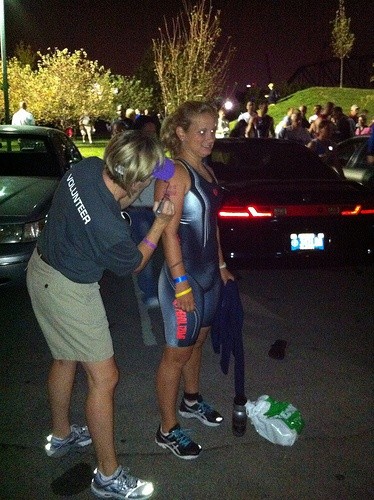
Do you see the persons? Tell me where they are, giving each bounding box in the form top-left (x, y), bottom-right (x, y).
top-left (215, 82), bottom-right (373, 155)
top-left (26, 130), bottom-right (177, 499)
top-left (80, 110), bottom-right (96, 144)
top-left (110, 104), bottom-right (162, 133)
top-left (12, 101), bottom-right (35, 126)
top-left (129, 116), bottom-right (161, 306)
top-left (156, 101), bottom-right (237, 460)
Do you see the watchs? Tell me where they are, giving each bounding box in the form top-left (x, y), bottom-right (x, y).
top-left (219, 262), bottom-right (227, 270)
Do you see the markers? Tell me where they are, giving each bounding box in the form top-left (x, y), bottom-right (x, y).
top-left (156, 194), bottom-right (169, 216)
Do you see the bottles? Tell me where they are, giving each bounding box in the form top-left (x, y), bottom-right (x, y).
top-left (232, 393), bottom-right (247, 437)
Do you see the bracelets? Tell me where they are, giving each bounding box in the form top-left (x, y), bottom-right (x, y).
top-left (142, 237), bottom-right (158, 249)
top-left (169, 259), bottom-right (183, 270)
top-left (171, 275), bottom-right (188, 284)
top-left (174, 287), bottom-right (192, 298)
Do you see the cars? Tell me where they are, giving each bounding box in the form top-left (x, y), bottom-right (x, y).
top-left (333, 134), bottom-right (374, 184)
top-left (202, 136), bottom-right (374, 270)
top-left (0, 124), bottom-right (82, 287)
